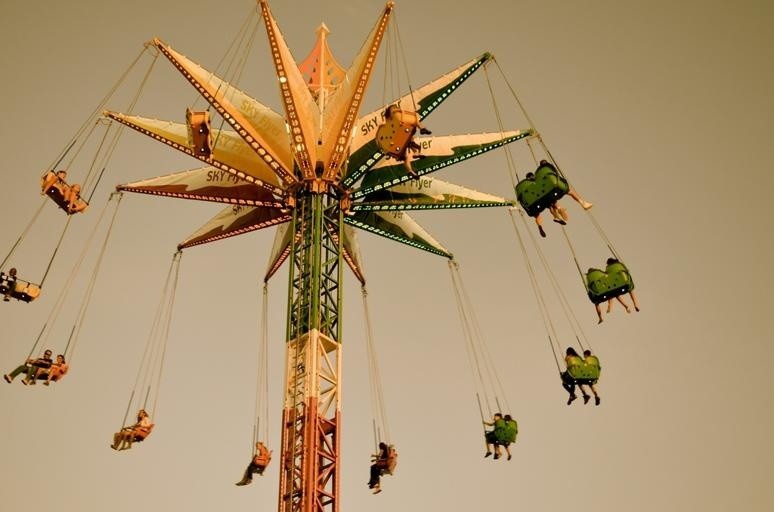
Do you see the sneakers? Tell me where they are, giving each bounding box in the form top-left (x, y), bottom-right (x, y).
top-left (583, 203), bottom-right (592, 210)
top-left (554, 207), bottom-right (568, 224)
top-left (408, 172), bottom-right (419, 179)
top-left (411, 142), bottom-right (421, 148)
top-left (568, 396), bottom-right (600, 405)
top-left (22, 380), bottom-right (28, 385)
top-left (485, 452), bottom-right (498, 459)
top-left (421, 129), bottom-right (432, 134)
top-left (538, 225), bottom-right (546, 237)
top-left (4, 374), bottom-right (12, 383)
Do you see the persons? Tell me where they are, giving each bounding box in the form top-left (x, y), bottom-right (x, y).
top-left (399, 145), bottom-right (426, 181)
top-left (577, 349), bottom-right (601, 406)
top-left (523, 172), bottom-right (567, 238)
top-left (539, 159), bottom-right (592, 221)
top-left (371, 445), bottom-right (396, 494)
top-left (560, 346), bottom-right (582, 406)
top-left (234, 441), bottom-right (268, 486)
top-left (28, 354), bottom-right (68, 386)
top-left (66, 184), bottom-right (88, 215)
top-left (39, 170), bottom-right (71, 208)
top-left (383, 104), bottom-right (432, 150)
top-left (0, 267), bottom-right (16, 302)
top-left (482, 412), bottom-right (505, 459)
top-left (584, 267), bottom-right (612, 325)
top-left (495, 414), bottom-right (517, 460)
top-left (3, 349), bottom-right (52, 385)
top-left (109, 409), bottom-right (149, 451)
top-left (605, 257), bottom-right (639, 313)
top-left (366, 442), bottom-right (391, 489)
